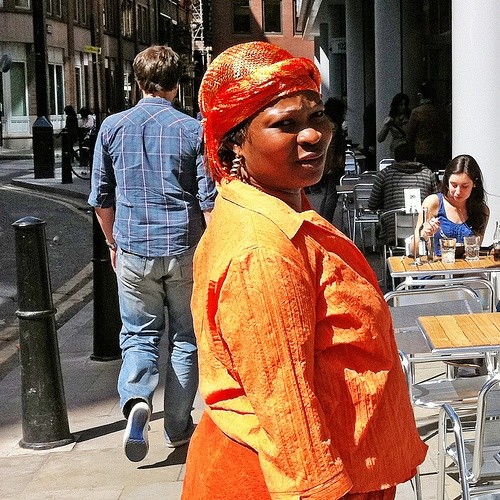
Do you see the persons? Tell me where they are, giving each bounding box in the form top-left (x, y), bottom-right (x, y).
top-left (368, 143), bottom-right (439, 291)
top-left (319, 97), bottom-right (348, 225)
top-left (62, 106), bottom-right (96, 167)
top-left (410, 155), bottom-right (490, 289)
top-left (180, 42), bottom-right (428, 500)
top-left (378, 81), bottom-right (440, 172)
top-left (87, 45), bottom-right (218, 462)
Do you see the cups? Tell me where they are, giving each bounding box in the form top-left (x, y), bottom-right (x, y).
top-left (439, 238), bottom-right (456, 264)
top-left (464, 237), bottom-right (480, 261)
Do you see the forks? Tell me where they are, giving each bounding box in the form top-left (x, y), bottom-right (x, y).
top-left (430, 212), bottom-right (447, 239)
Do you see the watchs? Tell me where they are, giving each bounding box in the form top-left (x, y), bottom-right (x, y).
top-left (419, 225), bottom-right (429, 242)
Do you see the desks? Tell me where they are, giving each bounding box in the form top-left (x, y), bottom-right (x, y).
top-left (417, 312), bottom-right (500, 380)
top-left (388, 254), bottom-right (500, 287)
top-left (347, 155), bottom-right (366, 159)
top-left (336, 185), bottom-right (376, 252)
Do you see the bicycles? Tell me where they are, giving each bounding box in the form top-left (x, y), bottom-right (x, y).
top-left (71, 126), bottom-right (95, 180)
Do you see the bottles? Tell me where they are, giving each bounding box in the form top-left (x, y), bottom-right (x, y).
top-left (493, 221), bottom-right (500, 262)
top-left (419, 207), bottom-right (435, 264)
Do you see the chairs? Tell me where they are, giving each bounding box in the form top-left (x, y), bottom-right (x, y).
top-left (340, 149), bottom-right (500, 500)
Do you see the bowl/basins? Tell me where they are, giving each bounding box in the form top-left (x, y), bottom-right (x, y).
top-left (456, 243), bottom-right (465, 258)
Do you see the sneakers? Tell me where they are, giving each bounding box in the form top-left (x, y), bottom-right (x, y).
top-left (124, 401), bottom-right (150, 462)
top-left (166, 423), bottom-right (198, 447)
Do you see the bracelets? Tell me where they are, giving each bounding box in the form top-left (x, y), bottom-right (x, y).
top-left (106, 241), bottom-right (116, 249)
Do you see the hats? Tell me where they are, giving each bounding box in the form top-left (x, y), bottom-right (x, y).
top-left (198, 42), bottom-right (323, 185)
